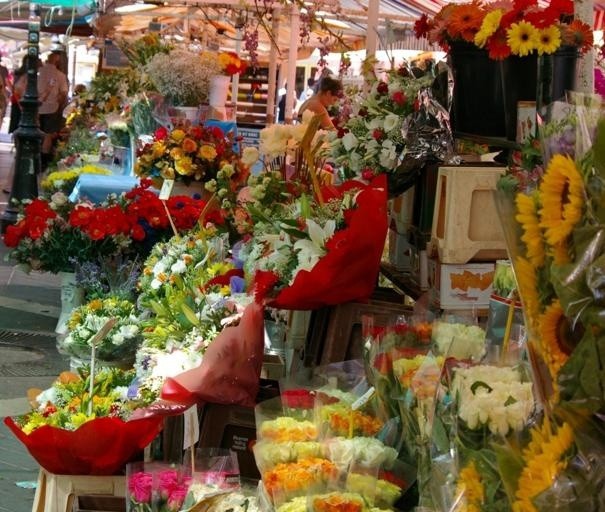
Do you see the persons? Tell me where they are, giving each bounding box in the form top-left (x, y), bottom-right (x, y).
top-left (314, 69), bottom-right (333, 94)
top-left (299, 79), bottom-right (315, 104)
top-left (13, 53), bottom-right (69, 165)
top-left (278, 84), bottom-right (297, 124)
top-left (72, 84), bottom-right (86, 97)
top-left (297, 76), bottom-right (344, 131)
top-left (8, 57), bottom-right (42, 147)
top-left (0, 54), bottom-right (13, 129)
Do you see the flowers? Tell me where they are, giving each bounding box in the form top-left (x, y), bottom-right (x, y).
top-left (5, 1), bottom-right (605, 508)
